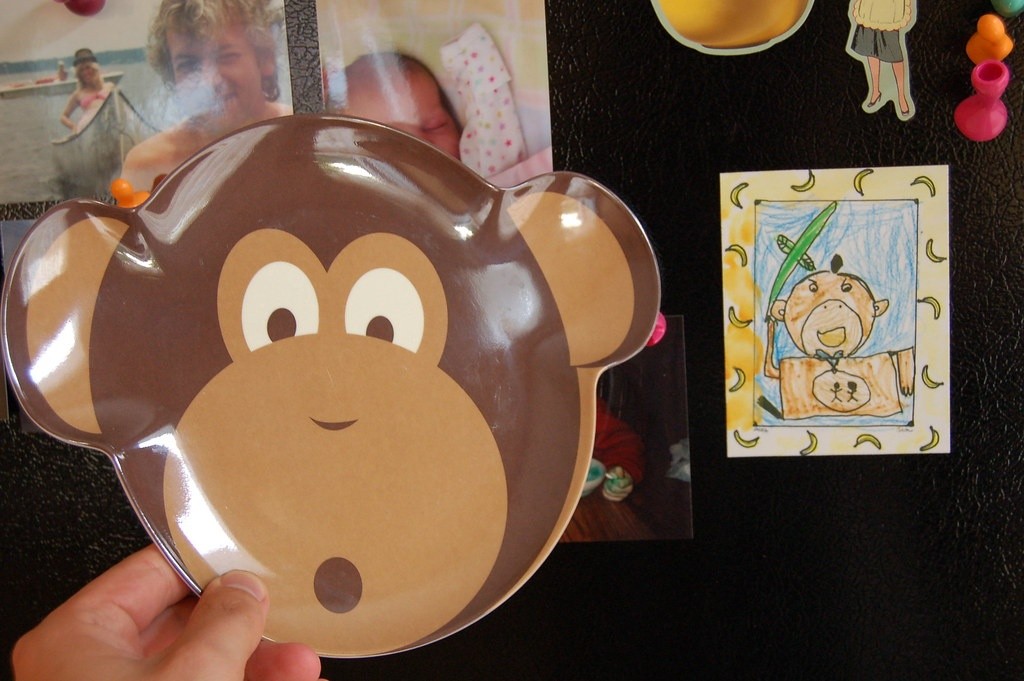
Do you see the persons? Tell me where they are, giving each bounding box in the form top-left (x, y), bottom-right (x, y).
top-left (330, 51), bottom-right (462, 165)
top-left (119, 1), bottom-right (294, 213)
top-left (59, 50), bottom-right (113, 132)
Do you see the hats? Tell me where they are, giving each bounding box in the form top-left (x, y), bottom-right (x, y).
top-left (73, 48), bottom-right (96, 66)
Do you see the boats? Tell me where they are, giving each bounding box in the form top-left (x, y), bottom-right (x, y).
top-left (1, 69), bottom-right (125, 99)
